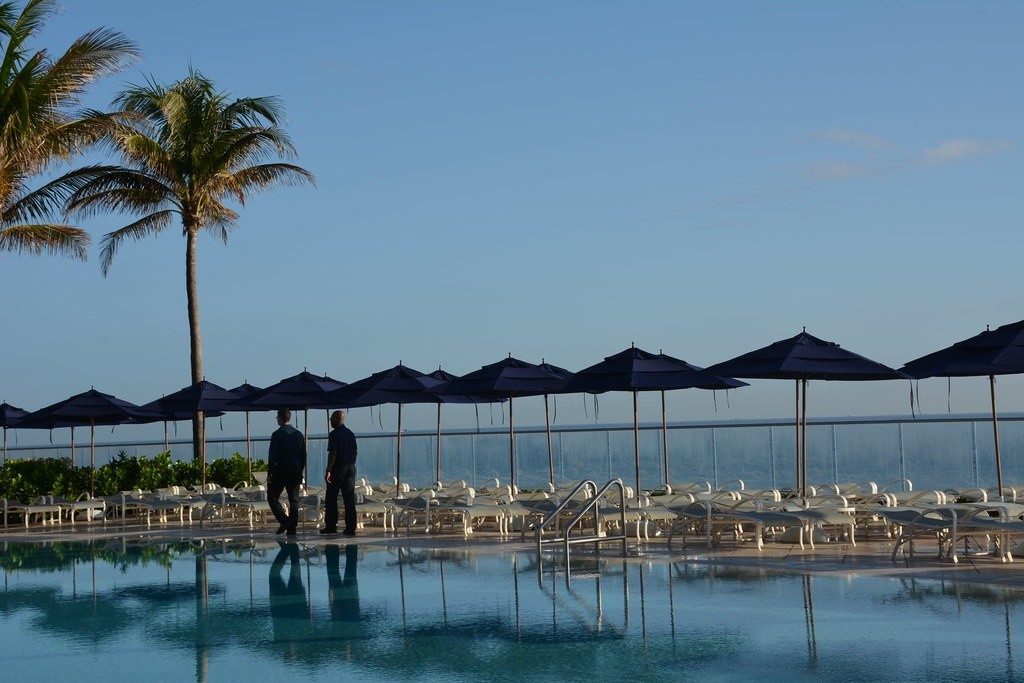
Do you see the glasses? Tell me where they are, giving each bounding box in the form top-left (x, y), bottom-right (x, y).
top-left (275, 417), bottom-right (279, 419)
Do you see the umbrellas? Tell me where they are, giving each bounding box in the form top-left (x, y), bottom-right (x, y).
top-left (0, 321), bottom-right (1024, 506)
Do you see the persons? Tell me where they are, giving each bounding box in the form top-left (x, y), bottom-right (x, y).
top-left (266, 411), bottom-right (307, 537)
top-left (265, 541), bottom-right (361, 646)
top-left (318, 411), bottom-right (358, 536)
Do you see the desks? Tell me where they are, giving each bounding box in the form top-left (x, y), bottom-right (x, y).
top-left (808, 505), bottom-right (856, 544)
top-left (965, 502), bottom-right (1024, 563)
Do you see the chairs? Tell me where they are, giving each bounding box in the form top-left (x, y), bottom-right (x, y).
top-left (0, 477), bottom-right (1024, 567)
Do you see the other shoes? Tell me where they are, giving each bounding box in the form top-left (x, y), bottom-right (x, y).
top-left (343, 529), bottom-right (355, 535)
top-left (277, 541), bottom-right (288, 547)
top-left (320, 527), bottom-right (336, 533)
top-left (276, 517), bottom-right (293, 534)
top-left (286, 528), bottom-right (296, 535)
